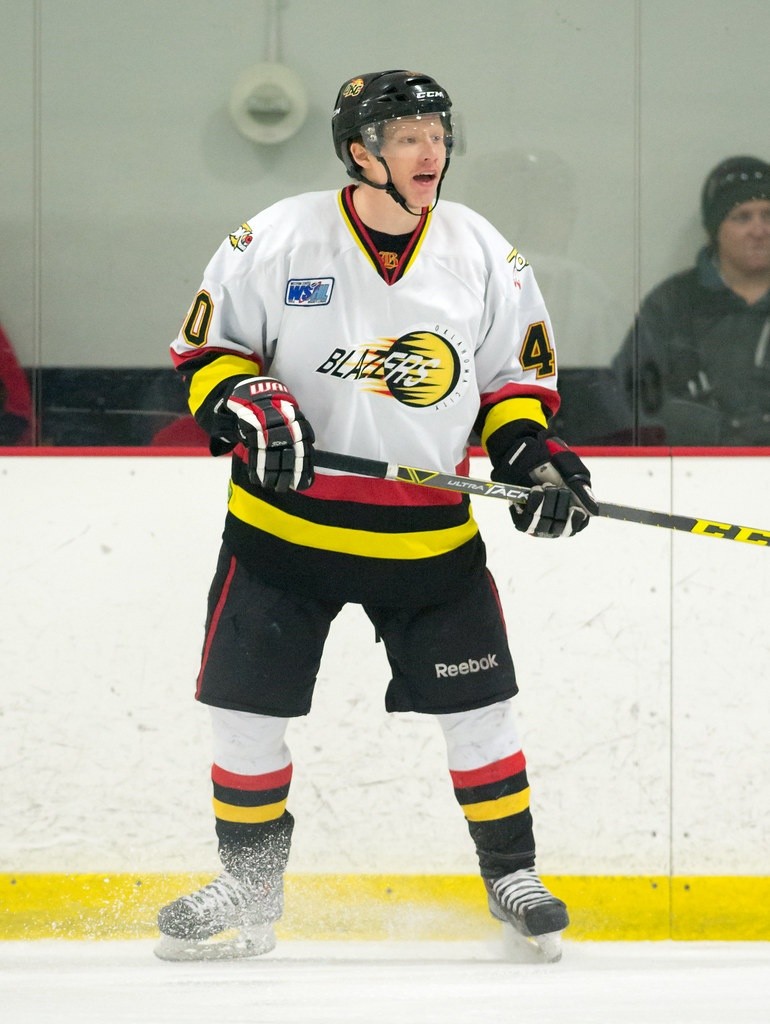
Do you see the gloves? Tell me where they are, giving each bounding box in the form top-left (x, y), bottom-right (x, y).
top-left (491, 428), bottom-right (600, 539)
top-left (224, 376), bottom-right (316, 495)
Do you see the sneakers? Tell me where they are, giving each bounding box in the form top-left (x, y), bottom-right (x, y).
top-left (470, 849), bottom-right (570, 963)
top-left (153, 810), bottom-right (295, 961)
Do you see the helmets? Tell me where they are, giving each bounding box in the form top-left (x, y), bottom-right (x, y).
top-left (331, 67), bottom-right (453, 176)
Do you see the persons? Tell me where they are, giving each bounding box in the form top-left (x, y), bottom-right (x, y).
top-left (152, 69), bottom-right (600, 968)
top-left (593, 149), bottom-right (770, 445)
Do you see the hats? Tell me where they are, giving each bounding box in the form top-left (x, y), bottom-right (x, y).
top-left (701, 155), bottom-right (770, 242)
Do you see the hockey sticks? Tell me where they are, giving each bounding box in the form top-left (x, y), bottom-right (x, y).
top-left (314, 449), bottom-right (770, 548)
